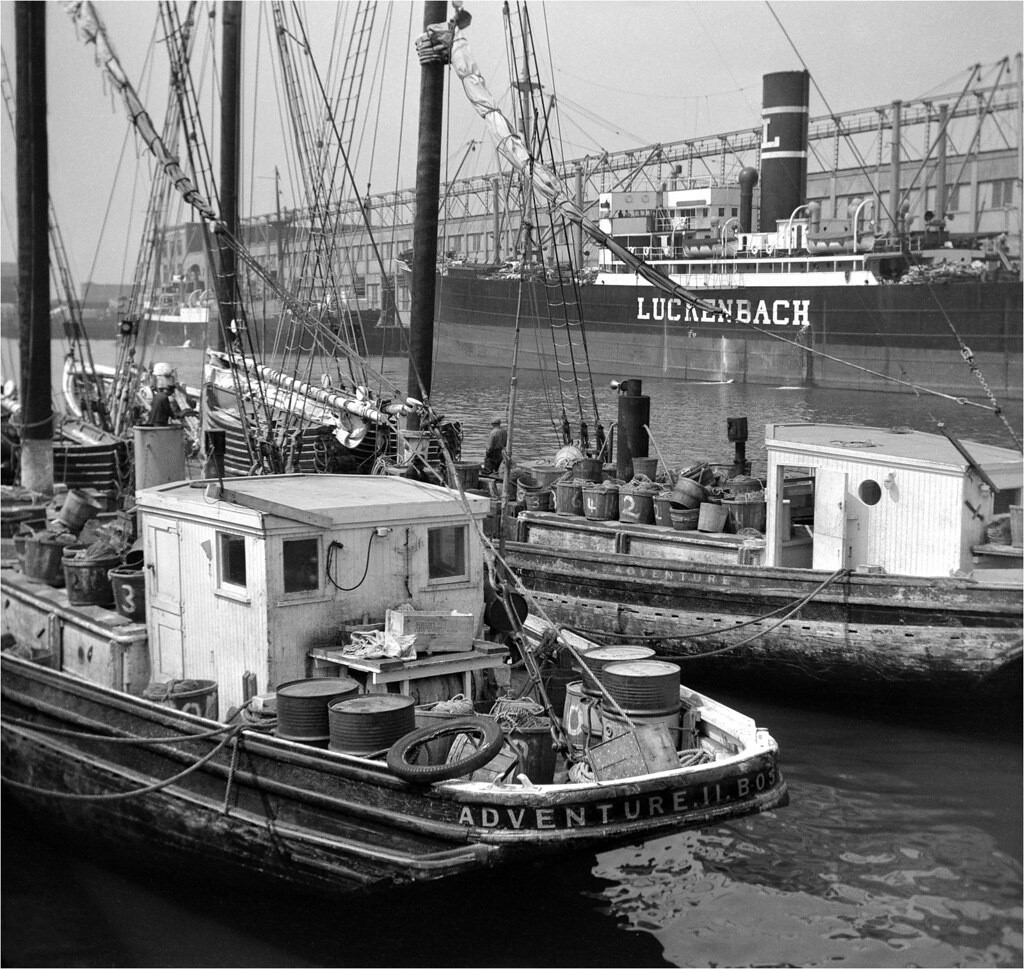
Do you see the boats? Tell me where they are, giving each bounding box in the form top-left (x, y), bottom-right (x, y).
top-left (395, 193), bottom-right (1023, 401)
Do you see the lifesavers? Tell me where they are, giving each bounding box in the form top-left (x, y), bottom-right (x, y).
top-left (384, 711), bottom-right (504, 786)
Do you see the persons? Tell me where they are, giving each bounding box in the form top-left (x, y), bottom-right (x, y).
top-left (147, 386), bottom-right (177, 427)
top-left (484, 419), bottom-right (507, 472)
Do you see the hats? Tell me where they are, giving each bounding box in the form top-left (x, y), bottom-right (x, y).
top-left (167, 385), bottom-right (176, 392)
top-left (491, 419), bottom-right (500, 424)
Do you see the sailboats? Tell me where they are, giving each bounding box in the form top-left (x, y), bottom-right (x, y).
top-left (61, 0), bottom-right (1023, 688)
top-left (1, 0), bottom-right (792, 909)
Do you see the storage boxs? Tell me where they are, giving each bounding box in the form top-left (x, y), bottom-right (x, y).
top-left (585, 721), bottom-right (678, 783)
top-left (386, 608), bottom-right (475, 654)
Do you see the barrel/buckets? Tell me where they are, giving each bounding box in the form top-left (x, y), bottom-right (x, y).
top-left (669, 476), bottom-right (709, 509)
top-left (110, 569), bottom-right (148, 621)
top-left (58, 489), bottom-right (102, 530)
top-left (449, 460), bottom-right (481, 490)
top-left (25, 537), bottom-right (64, 586)
top-left (572, 458), bottom-right (603, 484)
top-left (63, 542), bottom-right (96, 558)
top-left (124, 549), bottom-right (144, 567)
top-left (517, 476), bottom-right (543, 506)
top-left (557, 481), bottom-right (583, 516)
top-left (721, 496), bottom-right (767, 534)
top-left (618, 489), bottom-right (659, 524)
top-left (13, 531), bottom-right (33, 575)
top-left (530, 466), bottom-right (568, 489)
top-left (632, 457), bottom-right (658, 483)
top-left (62, 554), bottom-right (122, 606)
top-left (726, 476), bottom-right (758, 495)
top-left (582, 486), bottom-right (619, 521)
top-left (525, 490), bottom-right (551, 511)
top-left (146, 679), bottom-right (219, 722)
top-left (652, 494), bottom-right (673, 527)
top-left (697, 502), bottom-right (729, 533)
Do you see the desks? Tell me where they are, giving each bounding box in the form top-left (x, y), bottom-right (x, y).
top-left (313, 646), bottom-right (506, 703)
